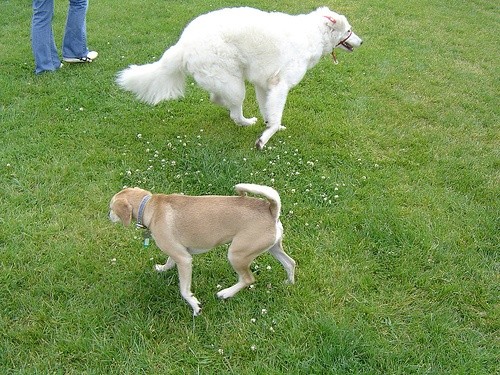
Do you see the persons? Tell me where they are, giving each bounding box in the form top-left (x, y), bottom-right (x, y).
top-left (31, 0), bottom-right (99, 75)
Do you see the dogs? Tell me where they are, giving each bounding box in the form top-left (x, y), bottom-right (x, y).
top-left (112, 6), bottom-right (363, 151)
top-left (108, 183), bottom-right (296, 317)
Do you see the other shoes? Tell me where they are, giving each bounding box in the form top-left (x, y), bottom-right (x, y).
top-left (59, 62), bottom-right (64, 68)
top-left (64, 50), bottom-right (98, 63)
